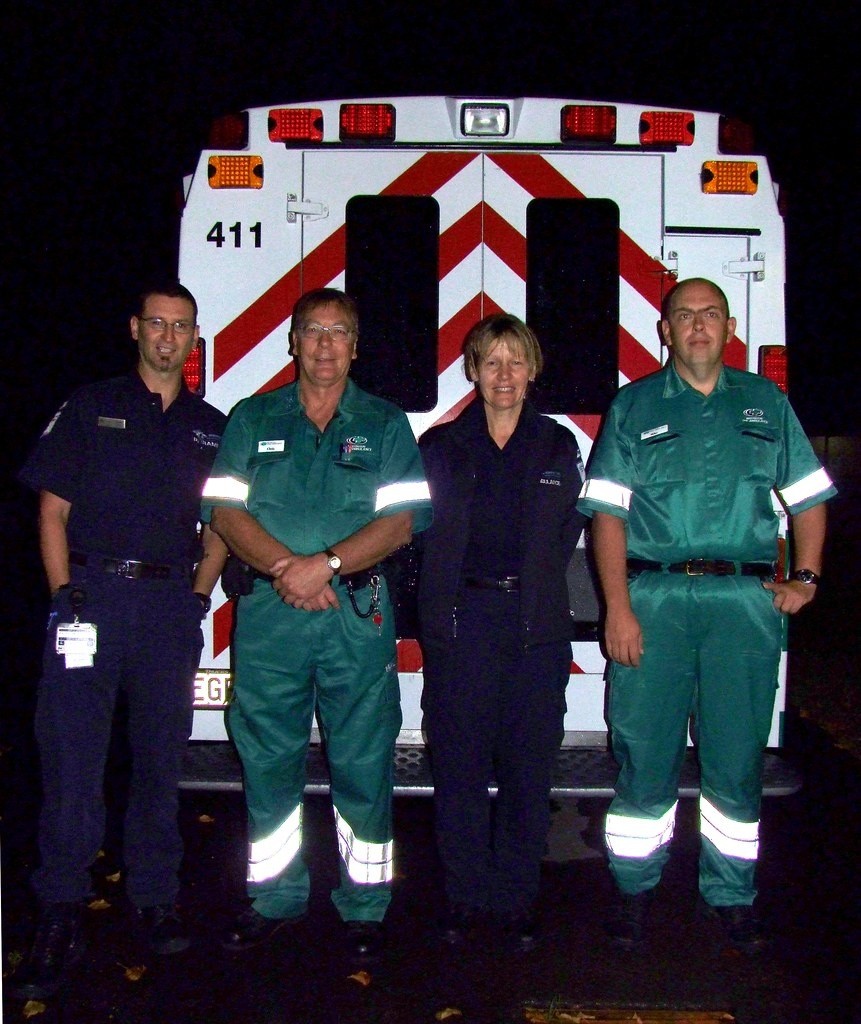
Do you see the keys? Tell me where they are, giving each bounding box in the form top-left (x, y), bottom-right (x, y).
top-left (371, 598), bottom-right (382, 636)
top-left (451, 619), bottom-right (457, 637)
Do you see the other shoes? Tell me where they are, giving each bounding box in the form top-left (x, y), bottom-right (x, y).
top-left (435, 904), bottom-right (485, 948)
top-left (612, 889), bottom-right (654, 944)
top-left (502, 919), bottom-right (549, 965)
top-left (40, 903), bottom-right (85, 953)
top-left (714, 905), bottom-right (763, 942)
top-left (131, 907), bottom-right (195, 957)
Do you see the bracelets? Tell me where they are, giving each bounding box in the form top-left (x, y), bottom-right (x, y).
top-left (195, 593), bottom-right (211, 613)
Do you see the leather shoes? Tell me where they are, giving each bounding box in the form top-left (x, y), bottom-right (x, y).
top-left (218, 909), bottom-right (302, 949)
top-left (346, 920), bottom-right (388, 965)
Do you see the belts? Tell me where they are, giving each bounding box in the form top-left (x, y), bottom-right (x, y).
top-left (252, 565), bottom-right (386, 586)
top-left (625, 557), bottom-right (776, 580)
top-left (67, 549), bottom-right (191, 581)
top-left (459, 575), bottom-right (522, 590)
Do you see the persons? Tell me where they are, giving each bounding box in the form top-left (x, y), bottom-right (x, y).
top-left (15, 283), bottom-right (231, 974)
top-left (576, 277), bottom-right (840, 939)
top-left (401, 313), bottom-right (585, 947)
top-left (200, 287), bottom-right (435, 953)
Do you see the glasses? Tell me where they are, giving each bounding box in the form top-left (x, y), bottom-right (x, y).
top-left (295, 323), bottom-right (358, 340)
top-left (138, 316), bottom-right (198, 334)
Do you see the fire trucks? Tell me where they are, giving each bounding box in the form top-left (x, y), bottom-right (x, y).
top-left (180, 96), bottom-right (803, 799)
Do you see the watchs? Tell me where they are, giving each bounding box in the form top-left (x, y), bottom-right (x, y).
top-left (324, 550), bottom-right (342, 575)
top-left (790, 569), bottom-right (819, 586)
